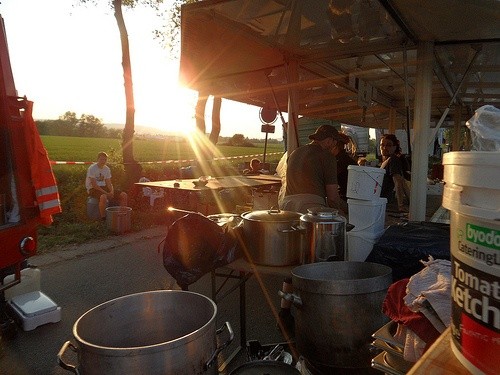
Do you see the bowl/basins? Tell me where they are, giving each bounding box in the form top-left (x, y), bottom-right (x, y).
top-left (192, 181), bottom-right (208, 186)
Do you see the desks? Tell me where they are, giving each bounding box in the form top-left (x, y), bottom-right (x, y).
top-left (211, 246), bottom-right (298, 373)
top-left (135, 174), bottom-right (281, 252)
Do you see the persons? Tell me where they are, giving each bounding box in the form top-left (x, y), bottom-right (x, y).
top-left (379, 134), bottom-right (410, 213)
top-left (333, 133), bottom-right (358, 207)
top-left (246, 158), bottom-right (274, 191)
top-left (357, 157), bottom-right (367, 166)
top-left (85, 152), bottom-right (128, 222)
top-left (278, 124), bottom-right (347, 214)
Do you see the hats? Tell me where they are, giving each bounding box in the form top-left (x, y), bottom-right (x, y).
top-left (308, 123), bottom-right (340, 140)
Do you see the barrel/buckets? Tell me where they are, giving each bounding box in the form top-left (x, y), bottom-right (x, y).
top-left (240, 205), bottom-right (304, 267)
top-left (229, 361), bottom-right (303, 375)
top-left (291, 206), bottom-right (356, 264)
top-left (346, 165), bottom-right (386, 201)
top-left (105, 206), bottom-right (133, 233)
top-left (440, 151), bottom-right (500, 375)
top-left (347, 197), bottom-right (388, 233)
top-left (56, 289), bottom-right (235, 375)
top-left (278, 261), bottom-right (393, 369)
top-left (346, 232), bottom-right (381, 262)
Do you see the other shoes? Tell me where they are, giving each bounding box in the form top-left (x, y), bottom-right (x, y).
top-left (99, 216), bottom-right (106, 224)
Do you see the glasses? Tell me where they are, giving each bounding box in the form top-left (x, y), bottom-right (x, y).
top-left (380, 143), bottom-right (394, 149)
top-left (255, 164), bottom-right (260, 167)
